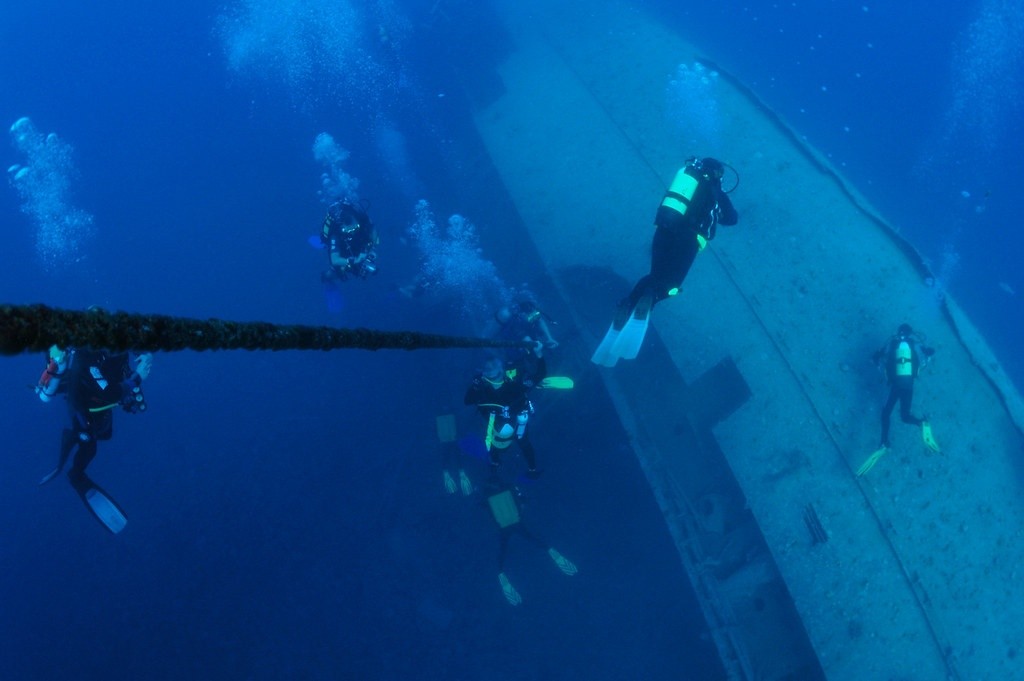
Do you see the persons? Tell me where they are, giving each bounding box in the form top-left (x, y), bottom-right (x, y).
top-left (37, 305), bottom-right (152, 535)
top-left (589, 157), bottom-right (739, 367)
top-left (857, 324), bottom-right (944, 476)
top-left (464, 353), bottom-right (574, 495)
top-left (321, 201), bottom-right (379, 283)
